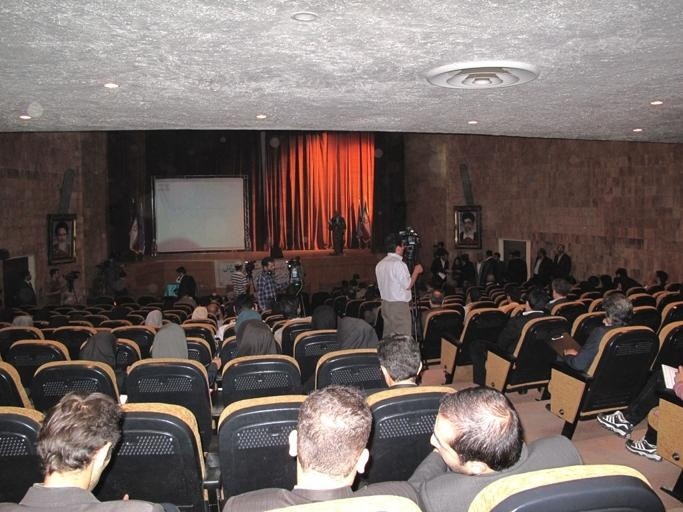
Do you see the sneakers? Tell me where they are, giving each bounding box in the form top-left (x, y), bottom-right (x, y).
top-left (596, 411), bottom-right (632, 438)
top-left (625, 438), bottom-right (662, 461)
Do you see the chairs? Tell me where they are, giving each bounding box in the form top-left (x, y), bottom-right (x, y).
top-left (0, 279), bottom-right (682, 512)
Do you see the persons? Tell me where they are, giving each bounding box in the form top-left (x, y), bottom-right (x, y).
top-left (329, 211), bottom-right (347, 256)
top-left (459, 212), bottom-right (478, 244)
top-left (44, 269), bottom-right (63, 305)
top-left (407, 386), bottom-right (583, 512)
top-left (175, 266), bottom-right (195, 297)
top-left (20, 270), bottom-right (36, 305)
top-left (11, 294), bottom-right (378, 394)
top-left (0, 391), bottom-right (166, 512)
top-left (335, 242), bottom-right (683, 461)
top-left (222, 385), bottom-right (425, 512)
top-left (232, 256), bottom-right (289, 310)
top-left (53, 223), bottom-right (70, 257)
top-left (112, 272), bottom-right (127, 296)
top-left (150, 238), bottom-right (158, 256)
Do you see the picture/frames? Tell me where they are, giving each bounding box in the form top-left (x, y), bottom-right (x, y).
top-left (46, 213), bottom-right (77, 265)
top-left (452, 205), bottom-right (482, 250)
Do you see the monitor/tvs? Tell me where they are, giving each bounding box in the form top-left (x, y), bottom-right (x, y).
top-left (166, 282), bottom-right (179, 297)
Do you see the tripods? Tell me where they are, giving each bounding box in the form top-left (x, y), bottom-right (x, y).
top-left (402, 246), bottom-right (429, 371)
top-left (289, 278), bottom-right (306, 317)
top-left (246, 275), bottom-right (256, 294)
top-left (63, 280), bottom-right (79, 304)
